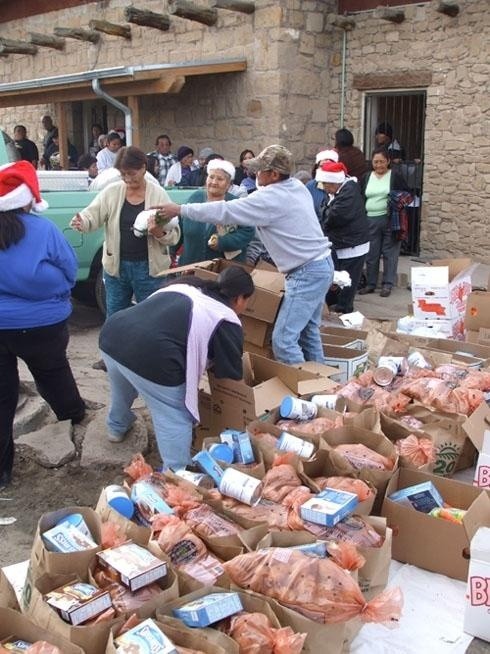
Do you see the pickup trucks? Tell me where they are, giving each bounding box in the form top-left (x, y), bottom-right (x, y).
top-left (0, 129), bottom-right (207, 315)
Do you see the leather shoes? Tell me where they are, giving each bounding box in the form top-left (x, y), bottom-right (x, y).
top-left (380, 288), bottom-right (391, 297)
top-left (359, 287), bottom-right (375, 294)
top-left (92, 360), bottom-right (105, 371)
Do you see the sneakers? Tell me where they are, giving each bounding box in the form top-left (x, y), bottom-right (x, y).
top-left (108, 426), bottom-right (134, 442)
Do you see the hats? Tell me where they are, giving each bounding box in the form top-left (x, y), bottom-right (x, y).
top-left (315, 162), bottom-right (350, 183)
top-left (0, 160), bottom-right (49, 213)
top-left (315, 147), bottom-right (339, 164)
top-left (178, 146), bottom-right (193, 161)
top-left (207, 158), bottom-right (235, 180)
top-left (77, 153), bottom-right (90, 166)
top-left (244, 144), bottom-right (293, 175)
top-left (376, 122), bottom-right (392, 138)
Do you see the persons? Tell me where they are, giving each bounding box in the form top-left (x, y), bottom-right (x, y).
top-left (146, 134), bottom-right (178, 186)
top-left (71, 147), bottom-right (180, 369)
top-left (146, 152), bottom-right (160, 186)
top-left (170, 159), bottom-right (255, 270)
top-left (165, 145), bottom-right (201, 187)
top-left (195, 148), bottom-right (213, 167)
top-left (295, 169), bottom-right (314, 185)
top-left (12, 126), bottom-right (39, 168)
top-left (0, 160), bottom-right (90, 490)
top-left (240, 162), bottom-right (258, 194)
top-left (149, 144), bottom-right (334, 367)
top-left (246, 230), bottom-right (274, 269)
top-left (90, 123), bottom-right (107, 158)
top-left (76, 153), bottom-right (100, 187)
top-left (44, 136), bottom-right (77, 170)
top-left (358, 148), bottom-right (411, 296)
top-left (317, 162), bottom-right (370, 313)
top-left (232, 148), bottom-right (258, 186)
top-left (98, 265), bottom-right (254, 474)
top-left (168, 154), bottom-right (223, 186)
top-left (41, 115), bottom-right (59, 170)
top-left (96, 133), bottom-right (124, 172)
top-left (305, 146), bottom-right (343, 226)
top-left (375, 123), bottom-right (408, 256)
top-left (334, 129), bottom-right (366, 186)
